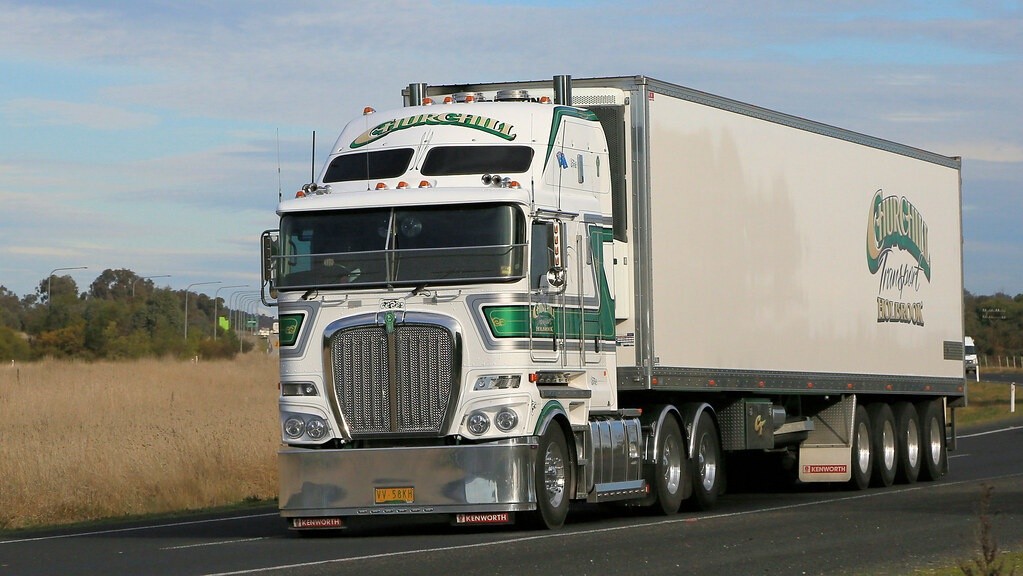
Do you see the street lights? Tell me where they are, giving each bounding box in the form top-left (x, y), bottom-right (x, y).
top-left (47, 266), bottom-right (88, 318)
top-left (213, 284), bottom-right (249, 342)
top-left (184, 281), bottom-right (222, 339)
top-left (228, 288), bottom-right (274, 354)
top-left (133, 274), bottom-right (173, 300)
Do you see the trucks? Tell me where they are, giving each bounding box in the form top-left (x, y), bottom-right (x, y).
top-left (964, 335), bottom-right (979, 374)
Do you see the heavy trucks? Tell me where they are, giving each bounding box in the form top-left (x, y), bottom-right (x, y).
top-left (258, 71), bottom-right (967, 529)
